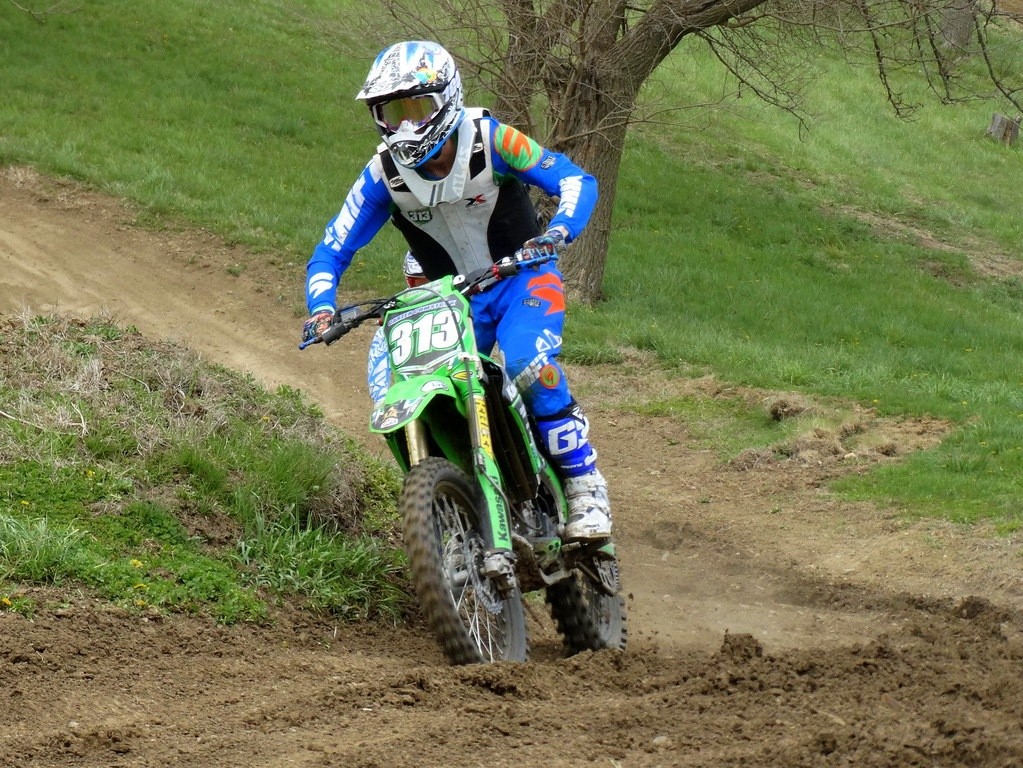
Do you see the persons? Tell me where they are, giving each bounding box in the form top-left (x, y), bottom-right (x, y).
top-left (304, 42), bottom-right (613, 538)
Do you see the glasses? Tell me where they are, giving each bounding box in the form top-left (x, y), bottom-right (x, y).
top-left (372, 91), bottom-right (443, 134)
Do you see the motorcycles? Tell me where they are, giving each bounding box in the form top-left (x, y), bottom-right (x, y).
top-left (298, 243), bottom-right (629, 663)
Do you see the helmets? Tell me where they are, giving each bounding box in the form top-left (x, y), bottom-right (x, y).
top-left (355, 41), bottom-right (465, 169)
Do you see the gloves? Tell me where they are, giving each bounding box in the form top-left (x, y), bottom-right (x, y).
top-left (522, 229), bottom-right (566, 272)
top-left (301, 310), bottom-right (343, 346)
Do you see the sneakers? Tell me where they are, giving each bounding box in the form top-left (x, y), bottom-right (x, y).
top-left (565, 467), bottom-right (613, 542)
